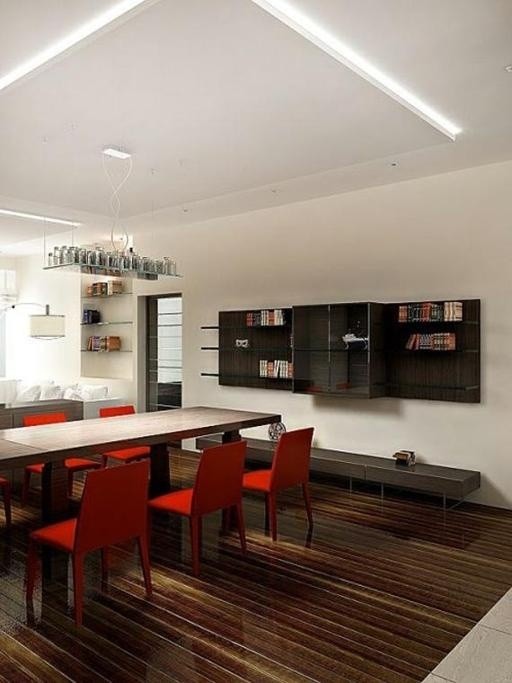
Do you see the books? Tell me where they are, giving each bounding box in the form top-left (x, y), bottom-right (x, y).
top-left (246, 310), bottom-right (287, 327)
top-left (87, 280), bottom-right (122, 298)
top-left (406, 333), bottom-right (455, 351)
top-left (399, 302), bottom-right (462, 323)
top-left (87, 336), bottom-right (120, 352)
top-left (83, 309), bottom-right (100, 325)
top-left (258, 360), bottom-right (293, 379)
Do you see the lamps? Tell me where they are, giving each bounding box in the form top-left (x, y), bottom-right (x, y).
top-left (0, 302), bottom-right (66, 340)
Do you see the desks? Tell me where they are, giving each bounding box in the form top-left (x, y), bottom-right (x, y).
top-left (0, 405), bottom-right (282, 570)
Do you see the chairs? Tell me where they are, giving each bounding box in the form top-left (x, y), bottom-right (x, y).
top-left (97, 405), bottom-right (149, 466)
top-left (241, 427), bottom-right (315, 548)
top-left (22, 411), bottom-right (100, 501)
top-left (145, 441), bottom-right (248, 577)
top-left (25, 457), bottom-right (153, 628)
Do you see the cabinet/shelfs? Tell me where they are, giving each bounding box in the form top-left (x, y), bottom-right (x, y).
top-left (194, 432), bottom-right (481, 513)
top-left (80, 293), bottom-right (133, 353)
top-left (199, 298), bottom-right (482, 403)
